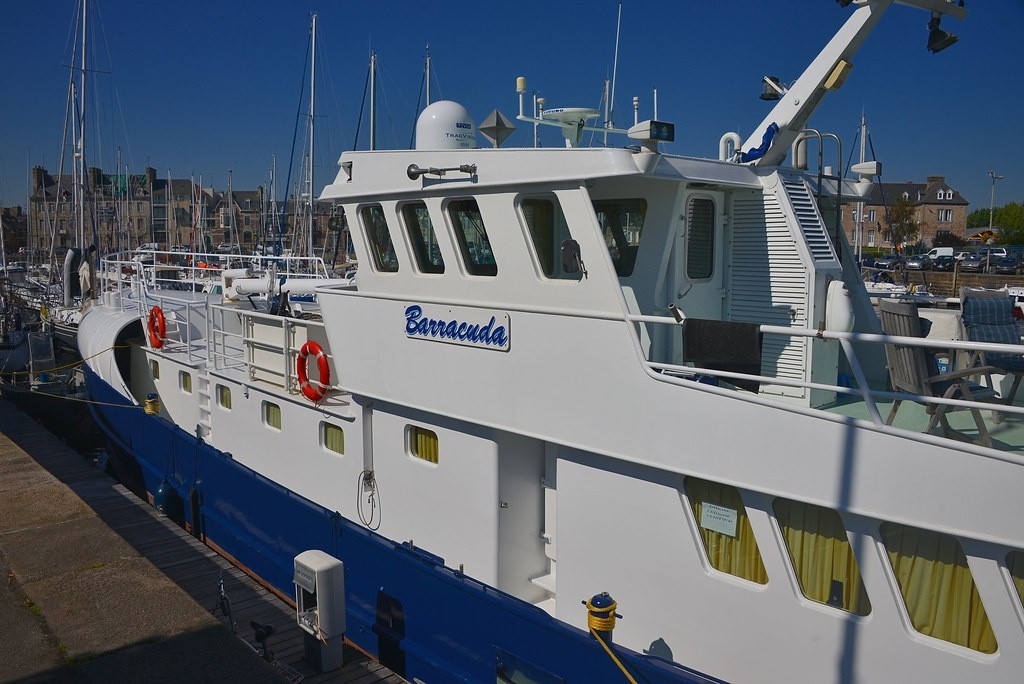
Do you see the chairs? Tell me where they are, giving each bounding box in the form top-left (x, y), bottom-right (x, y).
top-left (954, 286), bottom-right (1023, 424)
top-left (877, 298), bottom-right (1001, 450)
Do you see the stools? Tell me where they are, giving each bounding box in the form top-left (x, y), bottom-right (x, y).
top-left (837, 373), bottom-right (855, 398)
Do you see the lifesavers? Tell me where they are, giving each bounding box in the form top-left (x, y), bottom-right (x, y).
top-left (149, 305), bottom-right (166, 348)
top-left (297, 339), bottom-right (331, 402)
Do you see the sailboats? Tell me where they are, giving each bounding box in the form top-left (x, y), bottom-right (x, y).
top-left (0, 146), bottom-right (359, 358)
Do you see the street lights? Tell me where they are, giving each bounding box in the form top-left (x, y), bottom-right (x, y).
top-left (984, 171), bottom-right (1005, 273)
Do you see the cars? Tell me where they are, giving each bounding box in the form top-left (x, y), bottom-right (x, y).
top-left (920, 246), bottom-right (1024, 275)
top-left (904, 254), bottom-right (931, 270)
top-left (873, 255), bottom-right (906, 271)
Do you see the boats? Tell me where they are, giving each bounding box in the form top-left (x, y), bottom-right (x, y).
top-left (74, 0), bottom-right (1024, 684)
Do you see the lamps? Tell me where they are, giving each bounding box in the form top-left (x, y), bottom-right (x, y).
top-left (760, 74), bottom-right (782, 102)
top-left (850, 161), bottom-right (883, 185)
top-left (926, 13), bottom-right (960, 53)
top-left (628, 119), bottom-right (676, 153)
